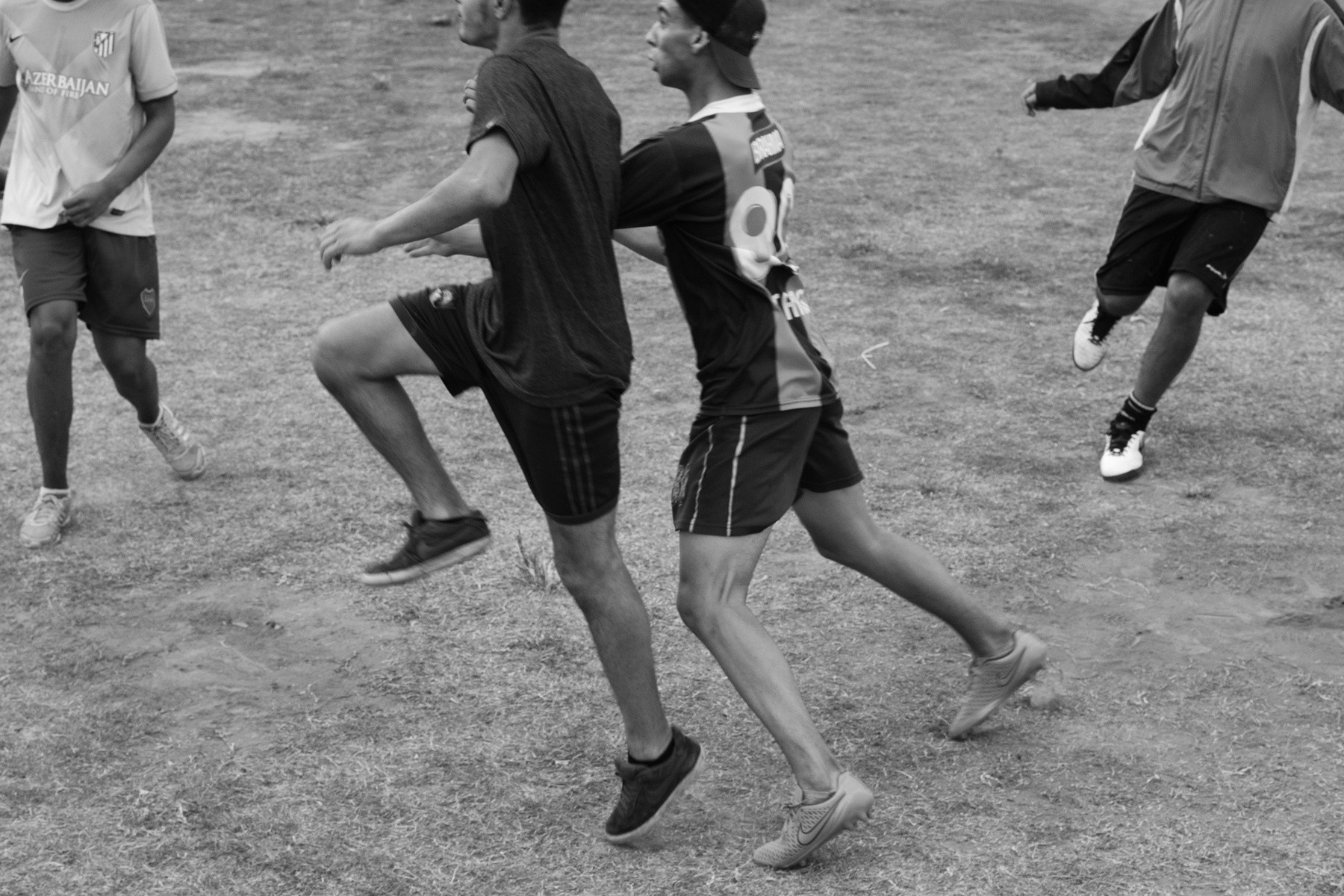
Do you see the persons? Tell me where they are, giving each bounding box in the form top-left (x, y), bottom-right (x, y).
top-left (465, 0), bottom-right (1046, 868)
top-left (0, 0), bottom-right (209, 553)
top-left (312, 0), bottom-right (703, 843)
top-left (1023, 0), bottom-right (1344, 483)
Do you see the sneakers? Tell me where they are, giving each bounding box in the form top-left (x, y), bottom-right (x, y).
top-left (19, 490), bottom-right (73, 549)
top-left (1073, 297), bottom-right (1123, 371)
top-left (604, 724), bottom-right (704, 843)
top-left (1099, 415), bottom-right (1146, 480)
top-left (948, 629), bottom-right (1048, 739)
top-left (135, 402), bottom-right (208, 480)
top-left (753, 769), bottom-right (875, 870)
top-left (359, 507), bottom-right (491, 585)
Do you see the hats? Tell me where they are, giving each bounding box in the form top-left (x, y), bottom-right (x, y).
top-left (676, 0), bottom-right (765, 89)
